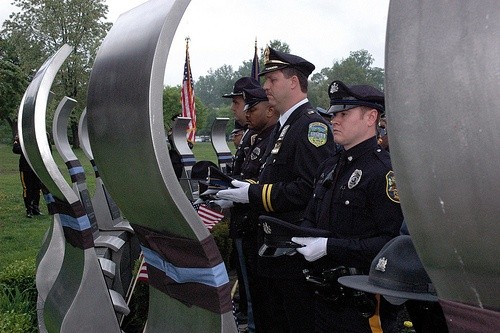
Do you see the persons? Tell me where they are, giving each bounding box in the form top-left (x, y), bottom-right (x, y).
top-left (12, 138), bottom-right (41, 217)
top-left (192, 48), bottom-right (448, 333)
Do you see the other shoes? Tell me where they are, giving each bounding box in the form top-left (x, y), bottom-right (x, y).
top-left (28, 212), bottom-right (33, 217)
top-left (33, 211), bottom-right (43, 215)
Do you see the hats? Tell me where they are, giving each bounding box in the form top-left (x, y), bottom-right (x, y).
top-left (258, 46), bottom-right (315, 78)
top-left (243, 87), bottom-right (269, 112)
top-left (326, 80), bottom-right (385, 114)
top-left (338, 235), bottom-right (441, 302)
top-left (257, 215), bottom-right (330, 257)
top-left (316, 107), bottom-right (333, 118)
top-left (222, 77), bottom-right (259, 97)
top-left (232, 120), bottom-right (248, 133)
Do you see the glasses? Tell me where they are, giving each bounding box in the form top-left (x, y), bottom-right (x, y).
top-left (231, 133), bottom-right (239, 139)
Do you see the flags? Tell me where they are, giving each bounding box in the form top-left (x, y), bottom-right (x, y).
top-left (137, 199), bottom-right (225, 283)
top-left (180, 49), bottom-right (197, 145)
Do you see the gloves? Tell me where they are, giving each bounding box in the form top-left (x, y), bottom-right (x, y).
top-left (209, 199), bottom-right (234, 208)
top-left (291, 236), bottom-right (328, 261)
top-left (383, 295), bottom-right (408, 305)
top-left (216, 179), bottom-right (250, 204)
